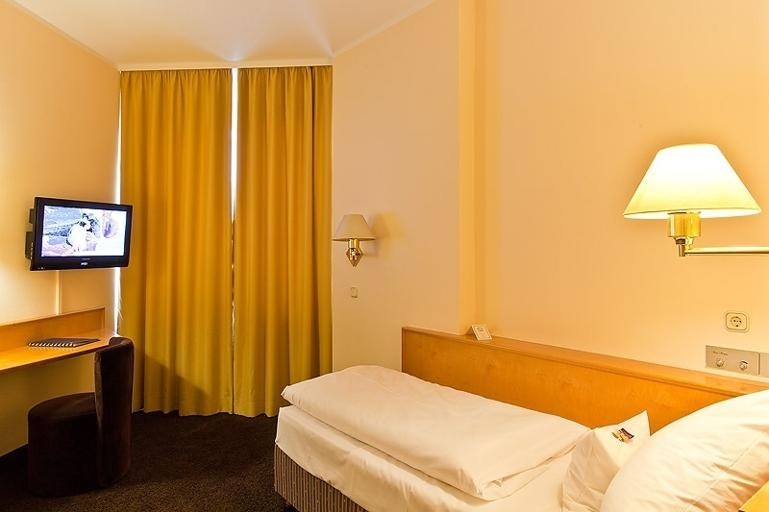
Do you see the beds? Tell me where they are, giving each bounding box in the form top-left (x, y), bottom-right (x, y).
top-left (273, 362), bottom-right (769, 512)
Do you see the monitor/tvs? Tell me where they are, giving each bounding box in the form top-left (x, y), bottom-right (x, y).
top-left (25, 197), bottom-right (132, 271)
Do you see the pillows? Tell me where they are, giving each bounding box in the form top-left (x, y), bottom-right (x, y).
top-left (559, 408), bottom-right (654, 512)
top-left (598, 388), bottom-right (769, 511)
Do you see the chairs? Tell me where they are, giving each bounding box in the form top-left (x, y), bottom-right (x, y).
top-left (25, 335), bottom-right (137, 494)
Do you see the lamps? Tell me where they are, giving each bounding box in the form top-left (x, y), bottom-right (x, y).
top-left (331, 212), bottom-right (377, 265)
top-left (621, 143), bottom-right (768, 257)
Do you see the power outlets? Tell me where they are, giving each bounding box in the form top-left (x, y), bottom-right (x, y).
top-left (724, 311), bottom-right (750, 333)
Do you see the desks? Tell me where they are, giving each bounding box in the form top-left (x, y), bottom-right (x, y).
top-left (0, 307), bottom-right (123, 378)
